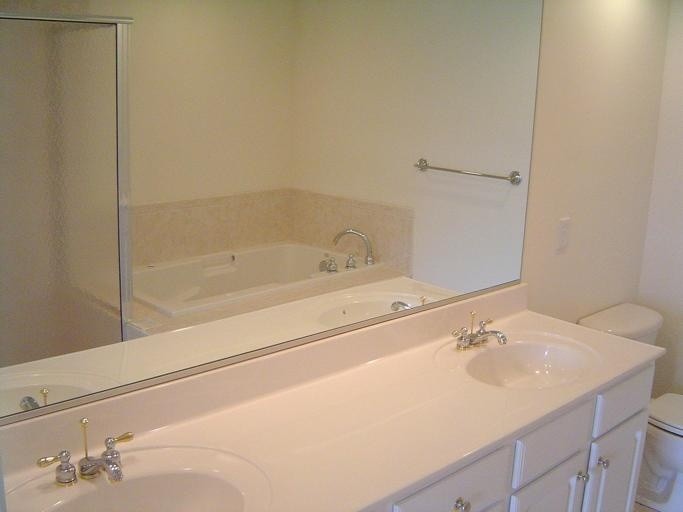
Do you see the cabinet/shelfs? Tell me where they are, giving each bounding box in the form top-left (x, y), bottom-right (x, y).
top-left (391, 443), bottom-right (510, 512)
top-left (507, 365), bottom-right (653, 512)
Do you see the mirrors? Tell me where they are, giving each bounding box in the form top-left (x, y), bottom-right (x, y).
top-left (0, 0), bottom-right (543, 427)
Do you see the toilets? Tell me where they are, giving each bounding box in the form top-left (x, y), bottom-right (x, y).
top-left (579, 302), bottom-right (683, 511)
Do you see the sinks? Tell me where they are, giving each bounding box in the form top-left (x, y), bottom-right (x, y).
top-left (36, 466), bottom-right (250, 512)
top-left (463, 340), bottom-right (586, 394)
top-left (0, 379), bottom-right (93, 420)
top-left (315, 293), bottom-right (442, 333)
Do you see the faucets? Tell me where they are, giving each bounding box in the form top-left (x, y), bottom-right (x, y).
top-left (452, 308), bottom-right (508, 352)
top-left (19, 386), bottom-right (51, 411)
top-left (78, 429), bottom-right (136, 483)
top-left (331, 228), bottom-right (374, 266)
top-left (390, 298), bottom-right (412, 312)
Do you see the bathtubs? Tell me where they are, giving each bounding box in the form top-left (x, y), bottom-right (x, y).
top-left (130, 239), bottom-right (386, 315)
